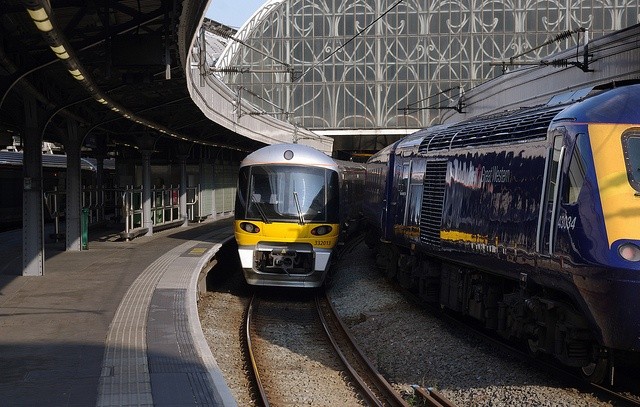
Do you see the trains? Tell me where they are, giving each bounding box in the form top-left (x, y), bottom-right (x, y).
top-left (235, 142), bottom-right (366, 295)
top-left (0, 151), bottom-right (117, 225)
top-left (365, 86), bottom-right (636, 385)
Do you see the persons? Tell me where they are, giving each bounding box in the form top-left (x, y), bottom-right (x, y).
top-left (152, 178), bottom-right (167, 223)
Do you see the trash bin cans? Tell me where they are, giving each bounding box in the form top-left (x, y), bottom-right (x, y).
top-left (80, 207), bottom-right (90, 248)
